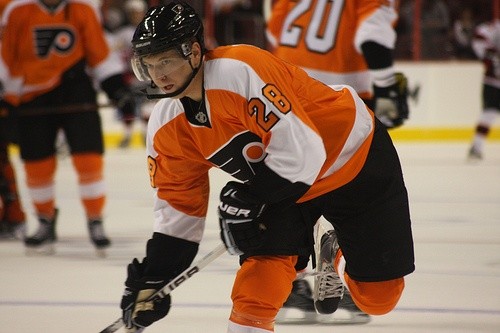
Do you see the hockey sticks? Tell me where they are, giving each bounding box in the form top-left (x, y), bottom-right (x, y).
top-left (96, 241), bottom-right (227, 333)
top-left (0, 101), bottom-right (120, 116)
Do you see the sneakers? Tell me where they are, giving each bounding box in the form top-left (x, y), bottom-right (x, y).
top-left (313, 218), bottom-right (344, 316)
top-left (24, 208), bottom-right (58, 256)
top-left (89, 220), bottom-right (111, 257)
top-left (282, 279), bottom-right (371, 324)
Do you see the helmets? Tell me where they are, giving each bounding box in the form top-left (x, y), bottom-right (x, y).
top-left (131, 0), bottom-right (204, 57)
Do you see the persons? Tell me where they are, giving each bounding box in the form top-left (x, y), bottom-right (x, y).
top-left (0, 0), bottom-right (500, 249)
top-left (120, 0), bottom-right (415, 333)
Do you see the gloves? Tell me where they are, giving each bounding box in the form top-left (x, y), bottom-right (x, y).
top-left (373, 73), bottom-right (408, 129)
top-left (217, 181), bottom-right (270, 256)
top-left (121, 258), bottom-right (175, 328)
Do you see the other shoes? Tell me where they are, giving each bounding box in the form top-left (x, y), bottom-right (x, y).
top-left (470, 139), bottom-right (486, 161)
